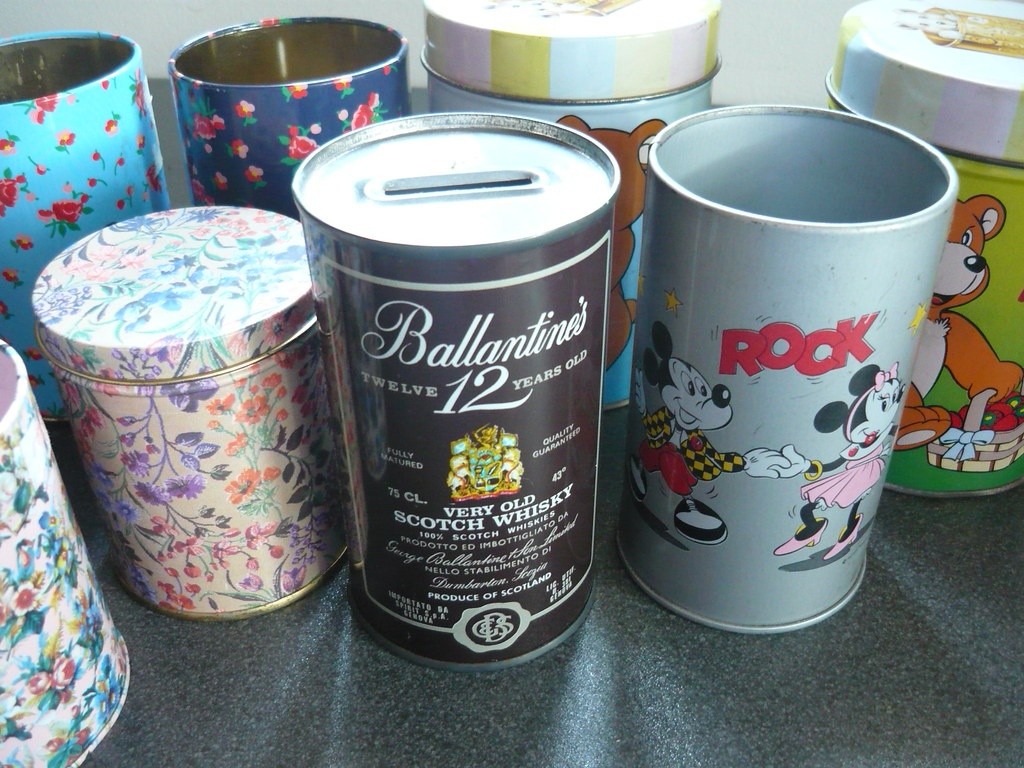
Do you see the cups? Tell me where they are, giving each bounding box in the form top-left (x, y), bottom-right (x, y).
top-left (421, 0), bottom-right (722, 413)
top-left (826, 0), bottom-right (1024, 498)
top-left (1, 30), bottom-right (171, 423)
top-left (167, 16), bottom-right (407, 221)
top-left (30, 207), bottom-right (347, 623)
top-left (1, 340), bottom-right (132, 767)
top-left (291, 109), bottom-right (621, 670)
top-left (614, 103), bottom-right (960, 635)
